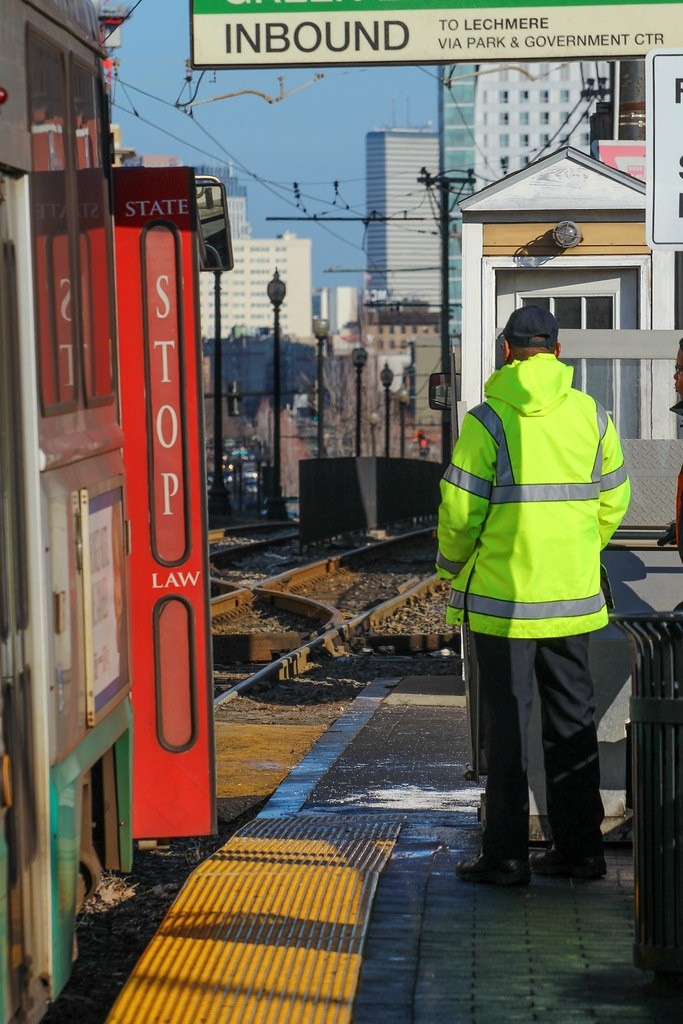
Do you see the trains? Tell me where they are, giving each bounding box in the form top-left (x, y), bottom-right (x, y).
top-left (3, 0), bottom-right (236, 1023)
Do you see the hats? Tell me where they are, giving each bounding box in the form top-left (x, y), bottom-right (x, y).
top-left (498, 305), bottom-right (558, 348)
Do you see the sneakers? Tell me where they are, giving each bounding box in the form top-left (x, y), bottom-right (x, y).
top-left (529, 843), bottom-right (606, 880)
top-left (455, 846), bottom-right (530, 887)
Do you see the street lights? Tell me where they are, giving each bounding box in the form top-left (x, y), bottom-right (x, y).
top-left (380, 367), bottom-right (392, 458)
top-left (349, 345), bottom-right (365, 456)
top-left (312, 315), bottom-right (329, 459)
top-left (399, 388), bottom-right (407, 460)
top-left (269, 266), bottom-right (288, 507)
top-left (417, 431), bottom-right (429, 456)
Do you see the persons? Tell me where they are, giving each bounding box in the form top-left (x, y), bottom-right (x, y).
top-left (435, 306), bottom-right (632, 887)
top-left (657, 339), bottom-right (683, 565)
